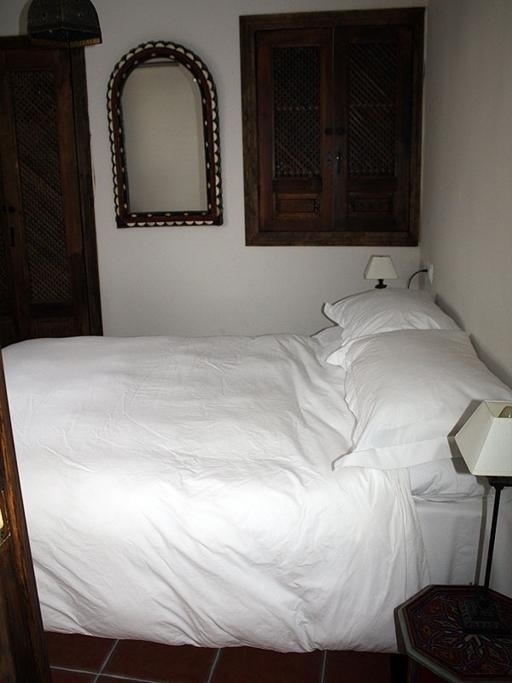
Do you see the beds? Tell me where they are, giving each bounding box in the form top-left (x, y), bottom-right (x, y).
top-left (2, 326), bottom-right (512, 652)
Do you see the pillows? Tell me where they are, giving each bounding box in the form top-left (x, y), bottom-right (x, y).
top-left (323, 288), bottom-right (512, 471)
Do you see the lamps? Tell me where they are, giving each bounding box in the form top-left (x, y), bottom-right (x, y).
top-left (27, 0), bottom-right (103, 49)
top-left (362, 253), bottom-right (399, 288)
top-left (454, 398), bottom-right (512, 634)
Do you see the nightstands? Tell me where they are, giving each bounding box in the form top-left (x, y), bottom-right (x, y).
top-left (393, 583), bottom-right (512, 683)
